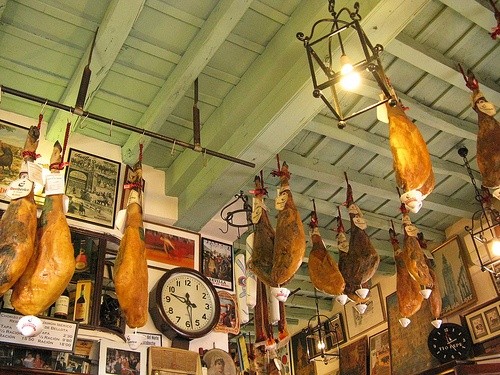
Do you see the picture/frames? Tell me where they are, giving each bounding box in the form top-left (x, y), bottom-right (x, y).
top-left (459, 295), bottom-right (500, 357)
top-left (343, 283), bottom-right (387, 341)
top-left (275, 340), bottom-right (293, 375)
top-left (339, 335), bottom-right (370, 375)
top-left (386, 291), bottom-right (442, 375)
top-left (0, 118), bottom-right (31, 204)
top-left (64, 147), bottom-right (121, 229)
top-left (291, 326), bottom-right (317, 375)
top-left (369, 328), bottom-right (391, 375)
top-left (98, 338), bottom-right (148, 375)
top-left (212, 291), bottom-right (240, 335)
top-left (201, 237), bottom-right (233, 291)
top-left (488, 262), bottom-right (500, 296)
top-left (142, 219), bottom-right (201, 273)
top-left (430, 234), bottom-right (478, 319)
top-left (311, 313), bottom-right (347, 356)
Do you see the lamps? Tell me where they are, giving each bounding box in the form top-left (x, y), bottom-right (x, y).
top-left (302, 288), bottom-right (341, 364)
top-left (457, 145), bottom-right (500, 273)
top-left (296, 0), bottom-right (397, 129)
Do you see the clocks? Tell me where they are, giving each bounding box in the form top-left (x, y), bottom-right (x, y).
top-left (149, 266), bottom-right (221, 340)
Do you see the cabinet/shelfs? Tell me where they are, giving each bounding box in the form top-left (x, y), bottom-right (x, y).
top-left (0, 209), bottom-right (127, 343)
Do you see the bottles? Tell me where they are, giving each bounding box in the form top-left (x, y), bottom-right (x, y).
top-left (2, 286), bottom-right (17, 312)
top-left (75, 239), bottom-right (88, 270)
top-left (75, 284), bottom-right (86, 322)
top-left (53, 288), bottom-right (70, 319)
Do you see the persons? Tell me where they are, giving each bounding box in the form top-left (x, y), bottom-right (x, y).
top-left (57, 357), bottom-right (81, 373)
top-left (114, 358), bottom-right (140, 375)
top-left (223, 304), bottom-right (235, 328)
top-left (213, 358), bottom-right (225, 375)
top-left (23, 353), bottom-right (45, 369)
